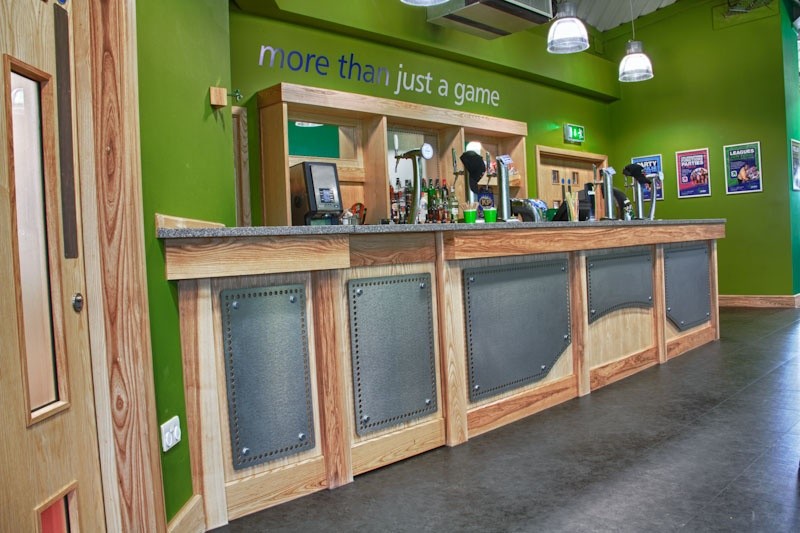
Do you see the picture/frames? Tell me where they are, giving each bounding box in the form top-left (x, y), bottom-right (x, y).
top-left (790, 138), bottom-right (800, 191)
top-left (631, 154), bottom-right (664, 202)
top-left (722, 140), bottom-right (763, 195)
top-left (675, 148), bottom-right (711, 199)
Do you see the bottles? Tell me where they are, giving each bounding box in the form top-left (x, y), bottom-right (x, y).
top-left (389, 177), bottom-right (459, 224)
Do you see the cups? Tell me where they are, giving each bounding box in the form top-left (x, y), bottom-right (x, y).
top-left (463, 211), bottom-right (477, 223)
top-left (483, 209), bottom-right (497, 223)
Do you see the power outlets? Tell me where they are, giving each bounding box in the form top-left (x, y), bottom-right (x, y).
top-left (159, 415), bottom-right (181, 453)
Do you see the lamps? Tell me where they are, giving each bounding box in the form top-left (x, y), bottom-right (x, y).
top-left (546, 0), bottom-right (590, 54)
top-left (620, 0), bottom-right (655, 83)
